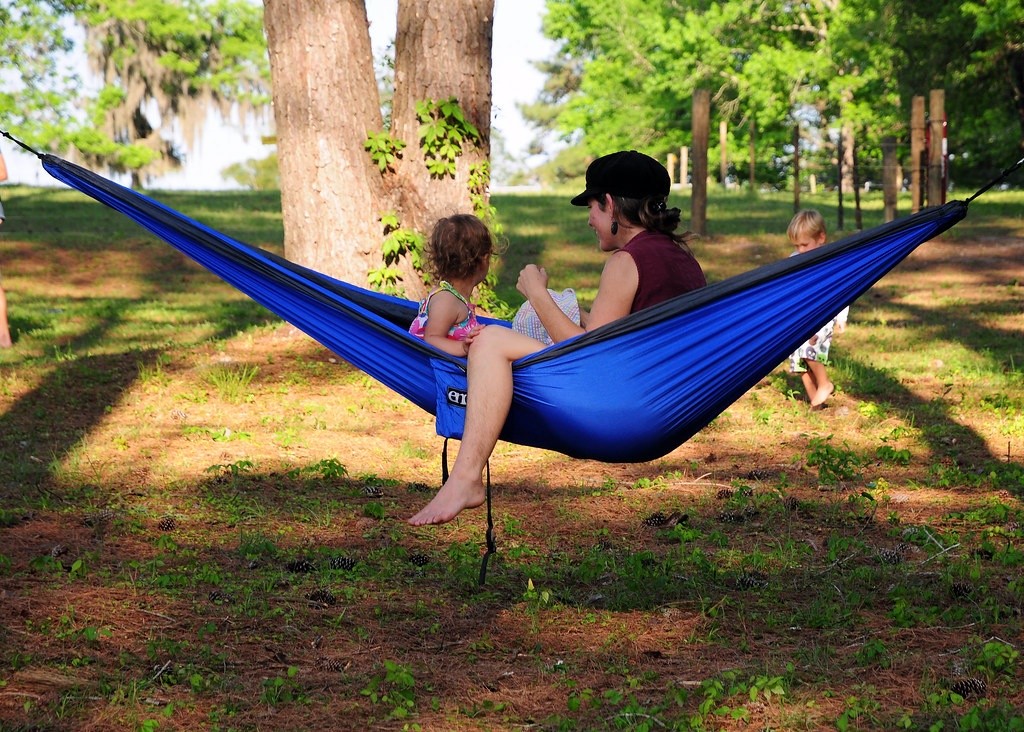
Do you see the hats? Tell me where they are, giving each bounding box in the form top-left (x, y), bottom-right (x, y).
top-left (571, 149), bottom-right (670, 206)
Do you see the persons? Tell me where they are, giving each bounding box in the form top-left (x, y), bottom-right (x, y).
top-left (408, 149), bottom-right (706, 526)
top-left (781, 208), bottom-right (847, 413)
top-left (408, 214), bottom-right (508, 358)
